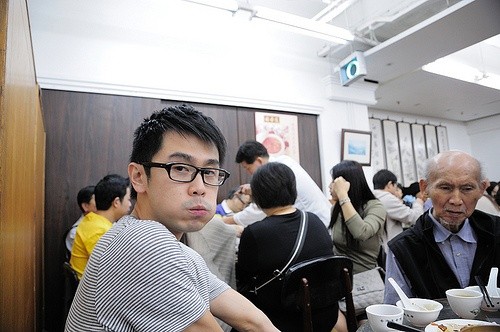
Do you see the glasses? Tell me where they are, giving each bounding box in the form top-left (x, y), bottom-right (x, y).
top-left (146, 162), bottom-right (230, 186)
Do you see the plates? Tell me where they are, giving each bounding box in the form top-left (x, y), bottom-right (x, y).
top-left (424, 318), bottom-right (494, 332)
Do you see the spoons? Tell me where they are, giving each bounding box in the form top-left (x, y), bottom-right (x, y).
top-left (484, 267), bottom-right (499, 296)
top-left (388, 277), bottom-right (426, 311)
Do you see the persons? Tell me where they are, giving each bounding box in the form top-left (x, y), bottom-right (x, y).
top-left (64, 185), bottom-right (97, 265)
top-left (326, 159), bottom-right (386, 332)
top-left (394, 178), bottom-right (500, 231)
top-left (231, 161), bottom-right (341, 332)
top-left (222, 138), bottom-right (334, 243)
top-left (178, 215), bottom-right (247, 332)
top-left (214, 183), bottom-right (252, 219)
top-left (69, 174), bottom-right (133, 283)
top-left (64, 105), bottom-right (282, 332)
top-left (382, 149), bottom-right (500, 307)
top-left (372, 168), bottom-right (426, 254)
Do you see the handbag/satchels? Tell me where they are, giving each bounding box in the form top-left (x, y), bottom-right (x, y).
top-left (338, 269), bottom-right (385, 315)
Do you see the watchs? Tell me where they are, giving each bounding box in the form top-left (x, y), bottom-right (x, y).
top-left (339, 196), bottom-right (351, 206)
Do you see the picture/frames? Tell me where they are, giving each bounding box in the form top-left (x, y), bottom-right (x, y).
top-left (340, 129), bottom-right (373, 167)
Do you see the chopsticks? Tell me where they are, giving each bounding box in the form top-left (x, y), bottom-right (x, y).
top-left (475, 276), bottom-right (494, 307)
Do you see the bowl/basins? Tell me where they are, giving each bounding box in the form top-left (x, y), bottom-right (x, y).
top-left (446, 289), bottom-right (483, 319)
top-left (396, 298), bottom-right (444, 328)
top-left (365, 304), bottom-right (404, 332)
top-left (460, 324), bottom-right (500, 332)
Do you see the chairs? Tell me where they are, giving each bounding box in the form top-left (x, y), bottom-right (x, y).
top-left (273, 256), bottom-right (358, 332)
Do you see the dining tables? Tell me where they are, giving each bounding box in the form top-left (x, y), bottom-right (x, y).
top-left (356, 298), bottom-right (500, 332)
